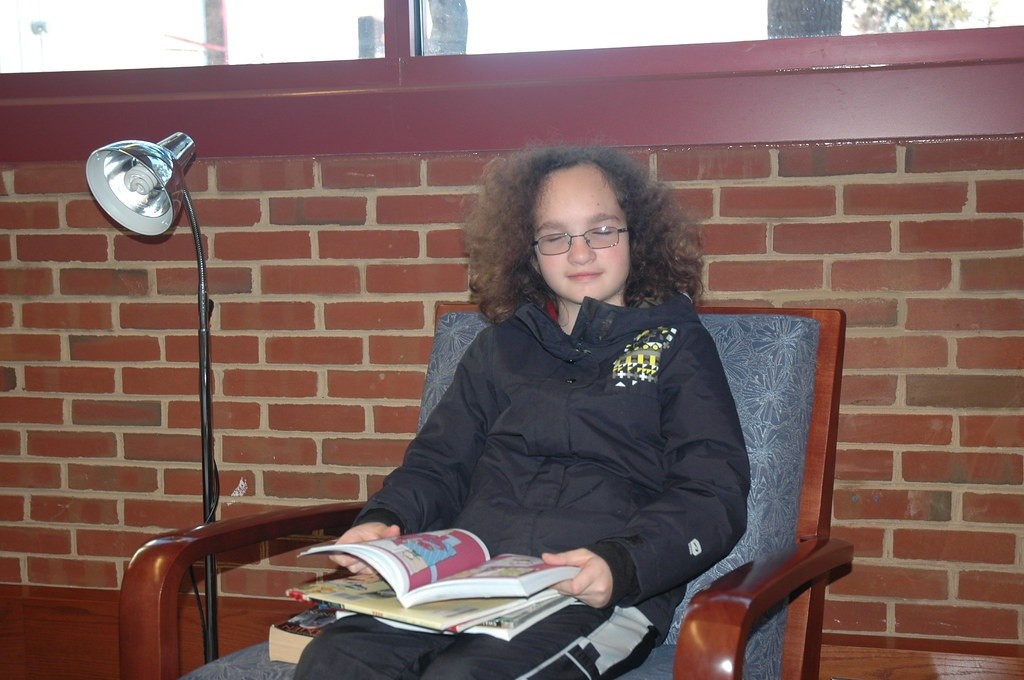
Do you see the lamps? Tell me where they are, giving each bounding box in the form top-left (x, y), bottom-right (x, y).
top-left (84, 132), bottom-right (219, 664)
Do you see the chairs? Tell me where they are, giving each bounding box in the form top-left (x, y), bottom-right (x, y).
top-left (117, 307), bottom-right (856, 680)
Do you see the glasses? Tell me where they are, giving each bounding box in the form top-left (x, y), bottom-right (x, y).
top-left (531, 226), bottom-right (632, 256)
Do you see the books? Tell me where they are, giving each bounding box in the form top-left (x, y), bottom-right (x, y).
top-left (287, 527), bottom-right (582, 642)
top-left (268, 605), bottom-right (338, 664)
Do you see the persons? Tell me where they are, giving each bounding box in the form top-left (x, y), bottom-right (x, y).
top-left (291, 138), bottom-right (751, 680)
